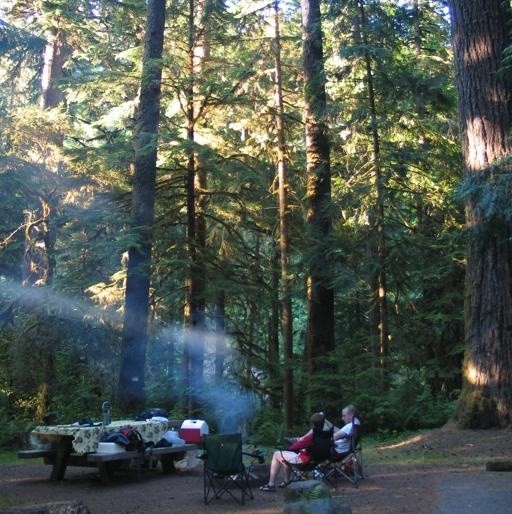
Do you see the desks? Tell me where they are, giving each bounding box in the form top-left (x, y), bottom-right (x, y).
top-left (30, 420), bottom-right (183, 481)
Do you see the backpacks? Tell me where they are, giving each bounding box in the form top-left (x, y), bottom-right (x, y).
top-left (120, 427), bottom-right (144, 453)
top-left (100, 428), bottom-right (130, 447)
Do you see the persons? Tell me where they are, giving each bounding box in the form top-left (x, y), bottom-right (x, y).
top-left (257, 412), bottom-right (325, 491)
top-left (283, 404), bottom-right (361, 480)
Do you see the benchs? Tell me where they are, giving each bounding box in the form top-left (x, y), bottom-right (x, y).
top-left (16, 443), bottom-right (200, 484)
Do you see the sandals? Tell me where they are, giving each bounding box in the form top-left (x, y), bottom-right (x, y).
top-left (278, 480), bottom-right (292, 489)
top-left (259, 483), bottom-right (276, 492)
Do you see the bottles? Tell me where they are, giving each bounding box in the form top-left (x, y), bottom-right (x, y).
top-left (102, 401), bottom-right (111, 426)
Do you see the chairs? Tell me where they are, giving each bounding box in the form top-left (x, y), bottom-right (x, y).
top-left (282, 415), bottom-right (364, 493)
top-left (195, 432), bottom-right (264, 506)
